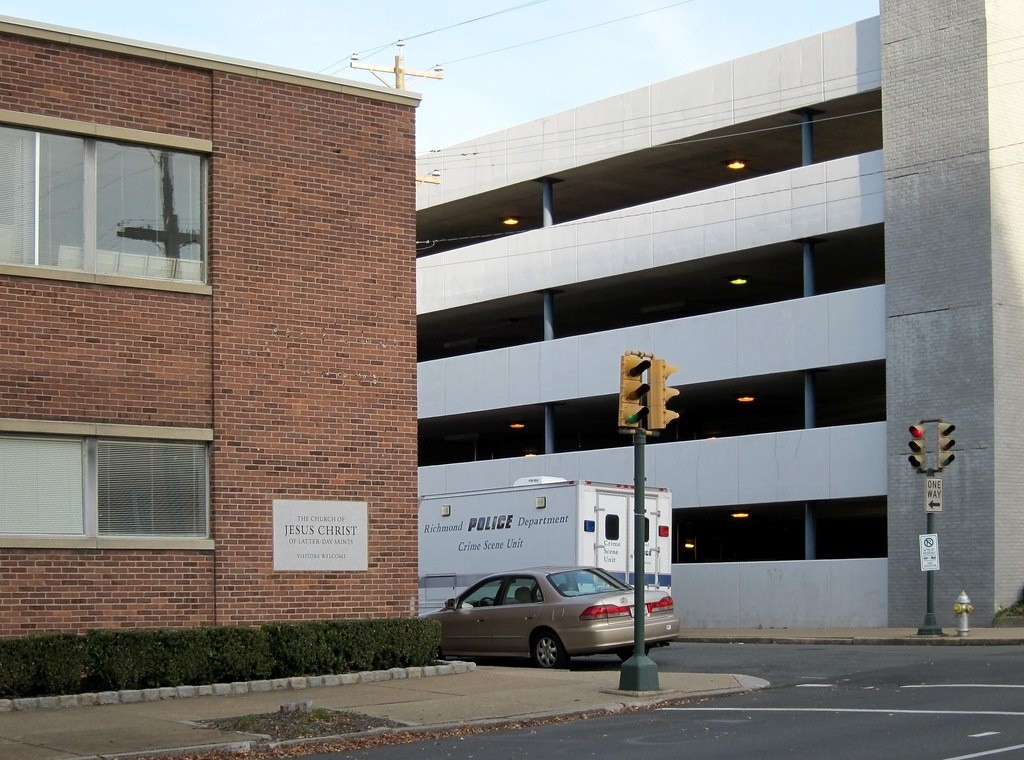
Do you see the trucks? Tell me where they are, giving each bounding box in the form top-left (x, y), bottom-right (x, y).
top-left (416, 475), bottom-right (671, 608)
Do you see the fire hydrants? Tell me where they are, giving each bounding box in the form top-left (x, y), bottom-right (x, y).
top-left (952, 590), bottom-right (973, 635)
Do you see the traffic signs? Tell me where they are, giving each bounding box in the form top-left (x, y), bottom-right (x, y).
top-left (924, 476), bottom-right (943, 513)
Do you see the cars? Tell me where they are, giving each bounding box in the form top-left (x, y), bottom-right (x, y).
top-left (422, 565), bottom-right (680, 670)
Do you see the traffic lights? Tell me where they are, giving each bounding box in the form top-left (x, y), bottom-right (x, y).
top-left (937, 423), bottom-right (955, 469)
top-left (909, 425), bottom-right (924, 469)
top-left (645, 358), bottom-right (680, 429)
top-left (618, 352), bottom-right (651, 431)
